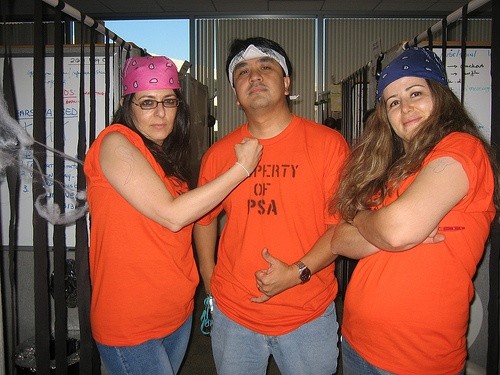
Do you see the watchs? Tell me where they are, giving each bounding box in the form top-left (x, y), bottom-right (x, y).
top-left (294, 260), bottom-right (312, 285)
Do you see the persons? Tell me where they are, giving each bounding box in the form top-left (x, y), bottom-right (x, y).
top-left (83, 57), bottom-right (263, 375)
top-left (191, 38), bottom-right (349, 374)
top-left (331, 49), bottom-right (494, 375)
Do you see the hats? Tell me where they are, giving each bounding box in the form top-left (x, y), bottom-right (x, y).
top-left (123, 56), bottom-right (180, 95)
top-left (376, 49), bottom-right (447, 102)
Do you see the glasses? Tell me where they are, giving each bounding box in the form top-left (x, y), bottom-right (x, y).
top-left (130, 98), bottom-right (179, 109)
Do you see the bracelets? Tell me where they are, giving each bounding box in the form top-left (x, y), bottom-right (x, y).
top-left (236, 161), bottom-right (250, 176)
